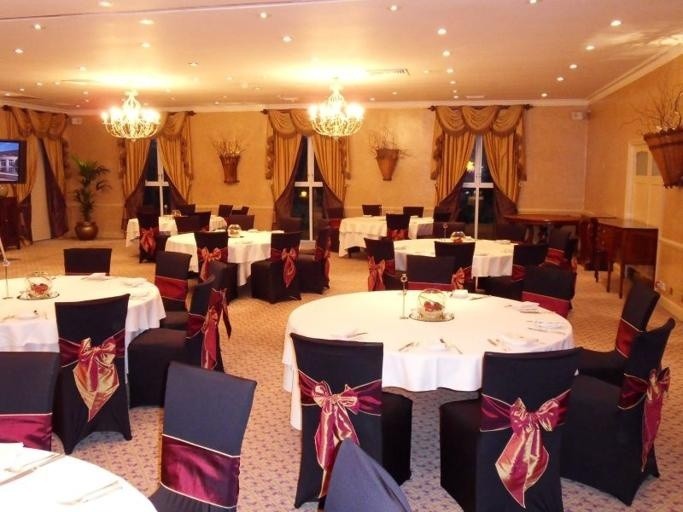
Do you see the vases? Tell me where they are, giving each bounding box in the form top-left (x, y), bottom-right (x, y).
top-left (375, 148), bottom-right (400, 181)
top-left (643, 125), bottom-right (683, 189)
top-left (219, 154), bottom-right (239, 183)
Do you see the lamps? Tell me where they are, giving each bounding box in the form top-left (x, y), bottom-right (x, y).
top-left (100, 90), bottom-right (162, 142)
top-left (307, 85), bottom-right (364, 142)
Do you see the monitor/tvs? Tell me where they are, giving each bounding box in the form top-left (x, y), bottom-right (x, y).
top-left (0, 139), bottom-right (28, 183)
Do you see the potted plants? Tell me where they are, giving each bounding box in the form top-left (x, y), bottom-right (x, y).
top-left (65, 151), bottom-right (112, 239)
